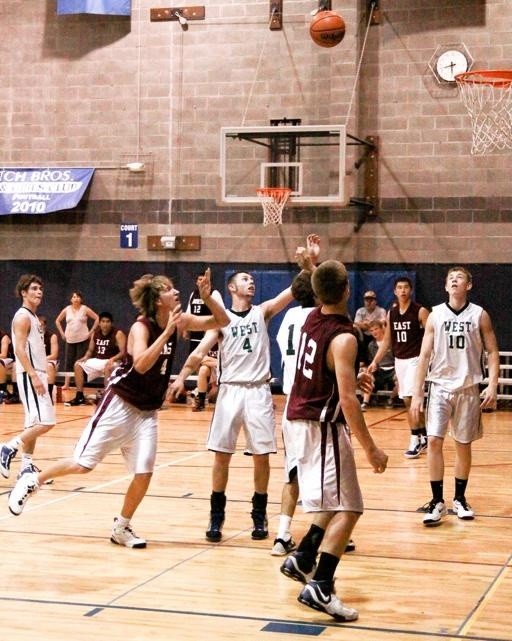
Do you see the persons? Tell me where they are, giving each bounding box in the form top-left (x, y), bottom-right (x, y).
top-left (279, 256), bottom-right (388, 625)
top-left (54, 288), bottom-right (99, 392)
top-left (0, 273), bottom-right (58, 486)
top-left (269, 246), bottom-right (356, 557)
top-left (182, 271), bottom-right (226, 355)
top-left (354, 290), bottom-right (387, 367)
top-left (408, 266), bottom-right (500, 526)
top-left (0, 328), bottom-right (14, 401)
top-left (367, 277), bottom-right (431, 459)
top-left (164, 232), bottom-right (322, 544)
top-left (6, 268), bottom-right (230, 550)
top-left (5, 313), bottom-right (61, 406)
top-left (360, 319), bottom-right (400, 410)
top-left (64, 311), bottom-right (127, 407)
top-left (191, 339), bottom-right (220, 414)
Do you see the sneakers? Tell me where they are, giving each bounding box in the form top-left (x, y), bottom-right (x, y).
top-left (17, 464), bottom-right (55, 490)
top-left (385, 399), bottom-right (394, 409)
top-left (192, 405), bottom-right (205, 412)
top-left (270, 533), bottom-right (297, 556)
top-left (0, 443), bottom-right (18, 479)
top-left (404, 434), bottom-right (429, 459)
top-left (252, 519), bottom-right (269, 538)
top-left (279, 550), bottom-right (336, 585)
top-left (452, 498), bottom-right (474, 519)
top-left (297, 578), bottom-right (359, 622)
top-left (0, 390), bottom-right (19, 404)
top-left (205, 516), bottom-right (225, 540)
top-left (422, 497), bottom-right (449, 525)
top-left (361, 402), bottom-right (368, 412)
top-left (110, 519), bottom-right (147, 548)
top-left (7, 472), bottom-right (39, 515)
top-left (64, 395), bottom-right (85, 406)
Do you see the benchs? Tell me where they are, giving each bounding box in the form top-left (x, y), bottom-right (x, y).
top-left (54, 369), bottom-right (278, 385)
top-left (352, 346), bottom-right (512, 407)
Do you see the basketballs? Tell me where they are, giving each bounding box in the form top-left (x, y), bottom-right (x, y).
top-left (309, 11), bottom-right (344, 45)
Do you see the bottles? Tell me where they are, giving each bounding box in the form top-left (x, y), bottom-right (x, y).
top-left (96, 390), bottom-right (102, 403)
top-left (52, 386), bottom-right (63, 404)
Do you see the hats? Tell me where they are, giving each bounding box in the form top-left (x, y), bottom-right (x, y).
top-left (363, 290), bottom-right (377, 298)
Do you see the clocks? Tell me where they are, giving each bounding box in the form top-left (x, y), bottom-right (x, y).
top-left (427, 41), bottom-right (475, 85)
